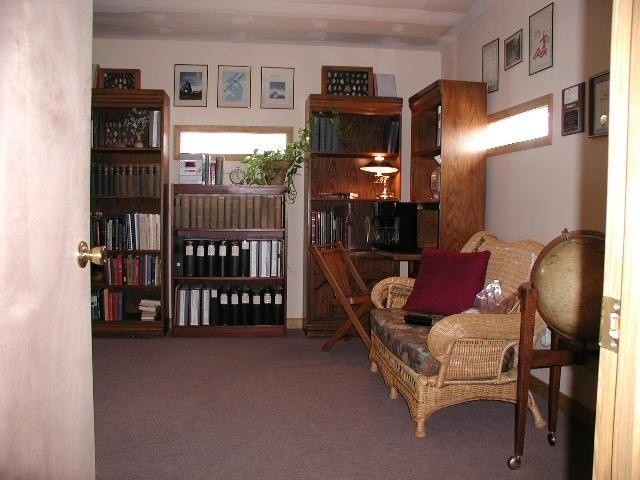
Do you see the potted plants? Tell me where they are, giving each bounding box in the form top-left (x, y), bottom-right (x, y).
top-left (240, 128), bottom-right (312, 204)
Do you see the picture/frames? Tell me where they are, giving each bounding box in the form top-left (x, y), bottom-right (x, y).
top-left (529, 2), bottom-right (554, 76)
top-left (504, 29), bottom-right (523, 71)
top-left (217, 65), bottom-right (251, 108)
top-left (260, 65), bottom-right (295, 110)
top-left (587, 70), bottom-right (610, 139)
top-left (481, 37), bottom-right (499, 94)
top-left (173, 63), bottom-right (208, 107)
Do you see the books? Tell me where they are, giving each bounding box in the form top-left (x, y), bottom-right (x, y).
top-left (310, 208), bottom-right (349, 250)
top-left (436, 104), bottom-right (442, 147)
top-left (90, 107), bottom-right (164, 325)
top-left (313, 114), bottom-right (400, 155)
top-left (169, 152), bottom-right (286, 329)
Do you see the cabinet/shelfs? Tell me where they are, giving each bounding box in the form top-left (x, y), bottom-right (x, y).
top-left (376, 80), bottom-right (488, 278)
top-left (171, 183), bottom-right (291, 338)
top-left (304, 94), bottom-right (403, 337)
top-left (90, 88), bottom-right (170, 339)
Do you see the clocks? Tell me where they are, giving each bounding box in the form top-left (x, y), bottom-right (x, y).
top-left (230, 167), bottom-right (245, 185)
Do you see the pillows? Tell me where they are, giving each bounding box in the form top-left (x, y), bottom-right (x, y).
top-left (402, 247), bottom-right (491, 316)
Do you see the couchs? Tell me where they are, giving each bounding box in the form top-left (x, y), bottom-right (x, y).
top-left (369, 231), bottom-right (552, 438)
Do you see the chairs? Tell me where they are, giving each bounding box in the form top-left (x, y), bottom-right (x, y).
top-left (309, 241), bottom-right (373, 352)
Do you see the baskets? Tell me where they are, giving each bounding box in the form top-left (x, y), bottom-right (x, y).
top-left (261, 160), bottom-right (293, 185)
